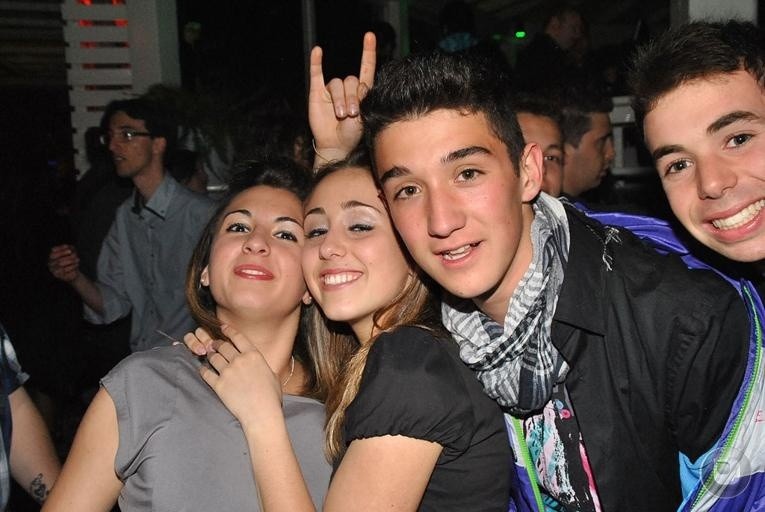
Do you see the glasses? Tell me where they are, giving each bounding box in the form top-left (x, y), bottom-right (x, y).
top-left (97, 131), bottom-right (158, 142)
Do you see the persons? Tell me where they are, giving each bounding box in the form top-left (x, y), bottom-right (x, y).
top-left (632, 22), bottom-right (765, 262)
top-left (44, 33), bottom-right (379, 511)
top-left (183, 16), bottom-right (615, 200)
top-left (168, 162), bottom-right (535, 511)
top-left (46, 96), bottom-right (220, 352)
top-left (0, 330), bottom-right (62, 512)
top-left (357, 54), bottom-right (765, 511)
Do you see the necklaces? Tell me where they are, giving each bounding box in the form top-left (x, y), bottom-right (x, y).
top-left (281, 355), bottom-right (295, 387)
